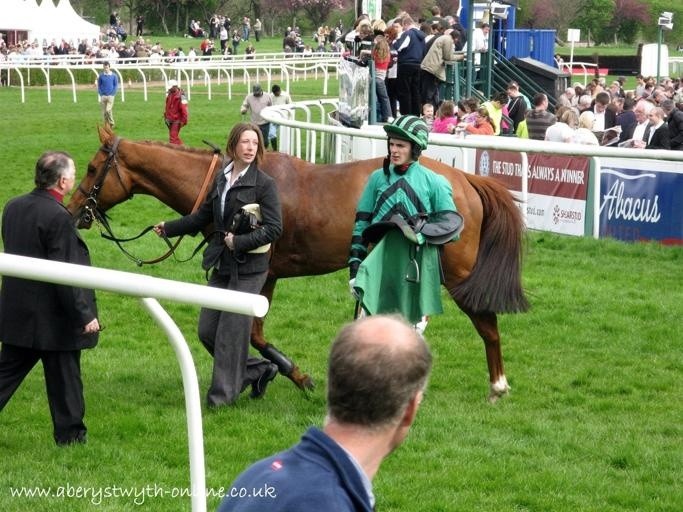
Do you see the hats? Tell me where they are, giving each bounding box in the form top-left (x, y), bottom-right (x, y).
top-left (507, 80), bottom-right (519, 90)
top-left (252, 84), bottom-right (262, 95)
top-left (166, 79), bottom-right (179, 91)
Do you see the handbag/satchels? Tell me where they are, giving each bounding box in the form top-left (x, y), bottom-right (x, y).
top-left (230, 202), bottom-right (272, 255)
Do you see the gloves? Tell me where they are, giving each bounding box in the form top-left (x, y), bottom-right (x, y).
top-left (348, 277), bottom-right (362, 302)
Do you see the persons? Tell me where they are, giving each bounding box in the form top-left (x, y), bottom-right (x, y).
top-left (212, 312), bottom-right (434, 512)
top-left (348, 116), bottom-right (463, 331)
top-left (1, 154), bottom-right (101, 445)
top-left (155, 122), bottom-right (277, 408)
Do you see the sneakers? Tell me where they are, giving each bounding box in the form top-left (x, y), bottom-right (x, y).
top-left (249, 362), bottom-right (280, 400)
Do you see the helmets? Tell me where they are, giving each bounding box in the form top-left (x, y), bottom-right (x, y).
top-left (382, 112), bottom-right (430, 152)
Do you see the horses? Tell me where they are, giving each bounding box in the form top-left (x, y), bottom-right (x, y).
top-left (65, 119), bottom-right (536, 406)
